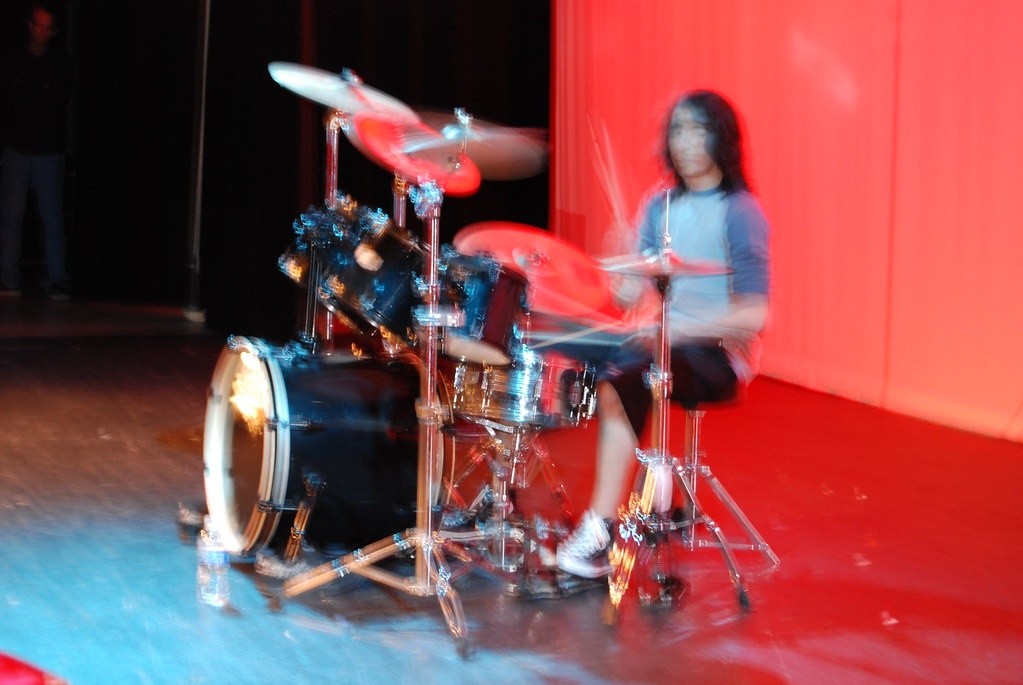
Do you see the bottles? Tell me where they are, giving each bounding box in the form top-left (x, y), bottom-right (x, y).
top-left (195, 522), bottom-right (228, 607)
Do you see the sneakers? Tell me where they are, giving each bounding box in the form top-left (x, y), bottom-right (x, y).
top-left (557, 507), bottom-right (620, 579)
top-left (441, 484), bottom-right (523, 541)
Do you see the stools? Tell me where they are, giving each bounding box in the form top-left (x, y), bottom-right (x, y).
top-left (637, 392), bottom-right (782, 612)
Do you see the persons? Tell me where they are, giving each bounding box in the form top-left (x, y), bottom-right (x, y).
top-left (499, 89), bottom-right (769, 599)
top-left (0, 7), bottom-right (78, 302)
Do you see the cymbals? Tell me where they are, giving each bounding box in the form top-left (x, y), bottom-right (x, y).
top-left (423, 117), bottom-right (551, 175)
top-left (351, 114), bottom-right (482, 196)
top-left (268, 61), bottom-right (425, 127)
top-left (601, 250), bottom-right (732, 285)
top-left (461, 227), bottom-right (611, 317)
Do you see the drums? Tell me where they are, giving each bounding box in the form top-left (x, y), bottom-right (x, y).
top-left (201, 335), bottom-right (458, 559)
top-left (451, 350), bottom-right (597, 430)
top-left (280, 197), bottom-right (429, 344)
top-left (429, 253), bottom-right (532, 368)
